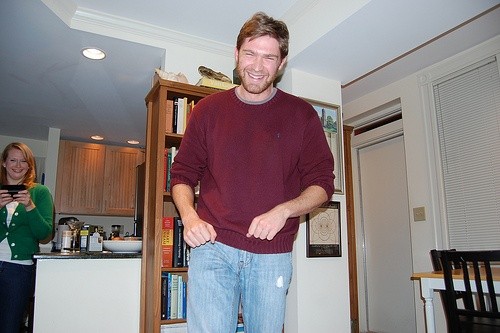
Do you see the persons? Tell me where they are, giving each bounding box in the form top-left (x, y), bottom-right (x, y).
top-left (0, 142), bottom-right (55, 333)
top-left (171, 12), bottom-right (335, 333)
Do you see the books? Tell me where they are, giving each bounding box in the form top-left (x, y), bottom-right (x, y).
top-left (159, 274), bottom-right (187, 320)
top-left (165, 147), bottom-right (200, 194)
top-left (166, 97), bottom-right (195, 134)
top-left (161, 215), bottom-right (190, 269)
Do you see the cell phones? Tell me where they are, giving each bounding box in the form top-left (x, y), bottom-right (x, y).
top-left (0, 184), bottom-right (26, 199)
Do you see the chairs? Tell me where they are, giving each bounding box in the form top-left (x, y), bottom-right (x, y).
top-left (430, 249), bottom-right (464, 301)
top-left (442, 250), bottom-right (500, 333)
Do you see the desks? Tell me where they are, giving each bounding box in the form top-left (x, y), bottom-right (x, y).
top-left (410, 267), bottom-right (500, 333)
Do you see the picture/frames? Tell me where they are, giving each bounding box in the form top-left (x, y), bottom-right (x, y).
top-left (300, 97), bottom-right (345, 195)
top-left (306, 201), bottom-right (342, 258)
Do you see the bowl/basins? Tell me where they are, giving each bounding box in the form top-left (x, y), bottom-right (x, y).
top-left (103, 240), bottom-right (142, 253)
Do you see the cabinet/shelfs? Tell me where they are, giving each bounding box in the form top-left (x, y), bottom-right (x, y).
top-left (54, 140), bottom-right (146, 217)
top-left (139, 79), bottom-right (245, 333)
